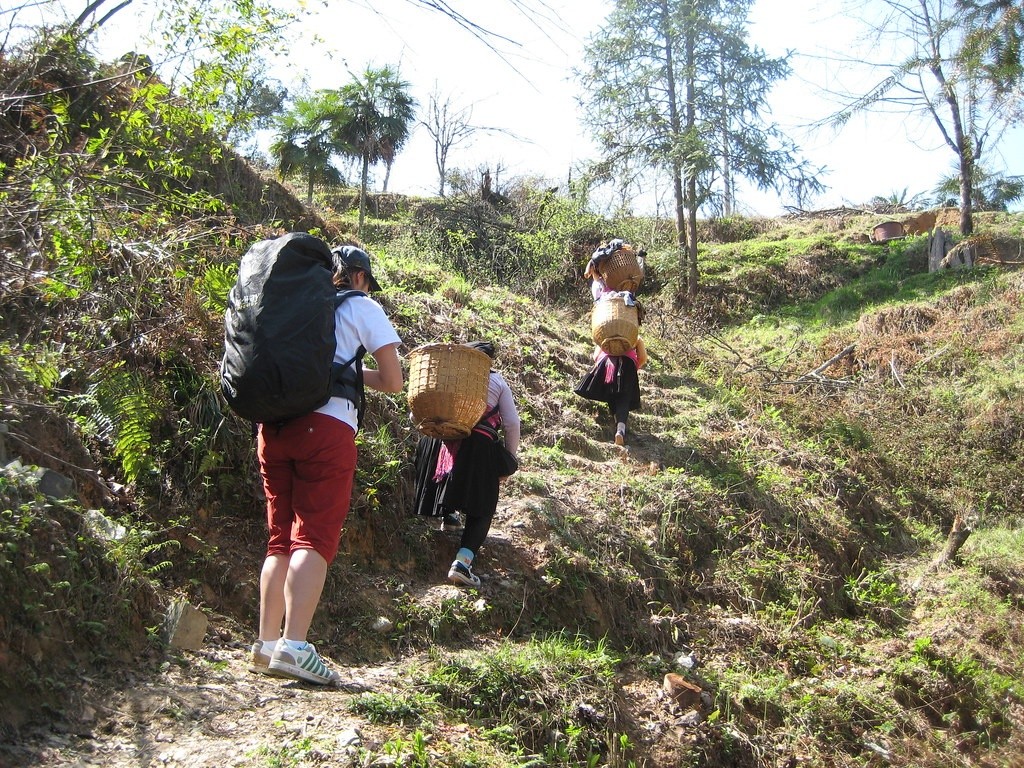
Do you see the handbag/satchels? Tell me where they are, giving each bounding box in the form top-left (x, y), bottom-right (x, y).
top-left (486, 443), bottom-right (518, 478)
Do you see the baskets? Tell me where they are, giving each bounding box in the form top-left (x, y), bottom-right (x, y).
top-left (409, 343), bottom-right (493, 441)
top-left (592, 297), bottom-right (639, 354)
top-left (598, 250), bottom-right (643, 292)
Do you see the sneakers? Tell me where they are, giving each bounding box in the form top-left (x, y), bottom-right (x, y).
top-left (268, 638), bottom-right (340, 686)
top-left (440, 514), bottom-right (465, 530)
top-left (448, 561), bottom-right (481, 587)
top-left (248, 639), bottom-right (273, 676)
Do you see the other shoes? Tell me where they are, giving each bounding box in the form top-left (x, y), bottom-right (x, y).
top-left (614, 430), bottom-right (624, 447)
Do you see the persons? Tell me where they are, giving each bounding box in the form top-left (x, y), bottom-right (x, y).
top-left (574, 243), bottom-right (647, 446)
top-left (413, 341), bottom-right (520, 586)
top-left (248, 245), bottom-right (402, 684)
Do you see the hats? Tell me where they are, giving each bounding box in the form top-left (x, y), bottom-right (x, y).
top-left (332, 245), bottom-right (382, 292)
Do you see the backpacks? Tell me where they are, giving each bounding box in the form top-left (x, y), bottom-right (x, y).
top-left (221, 232), bottom-right (369, 430)
